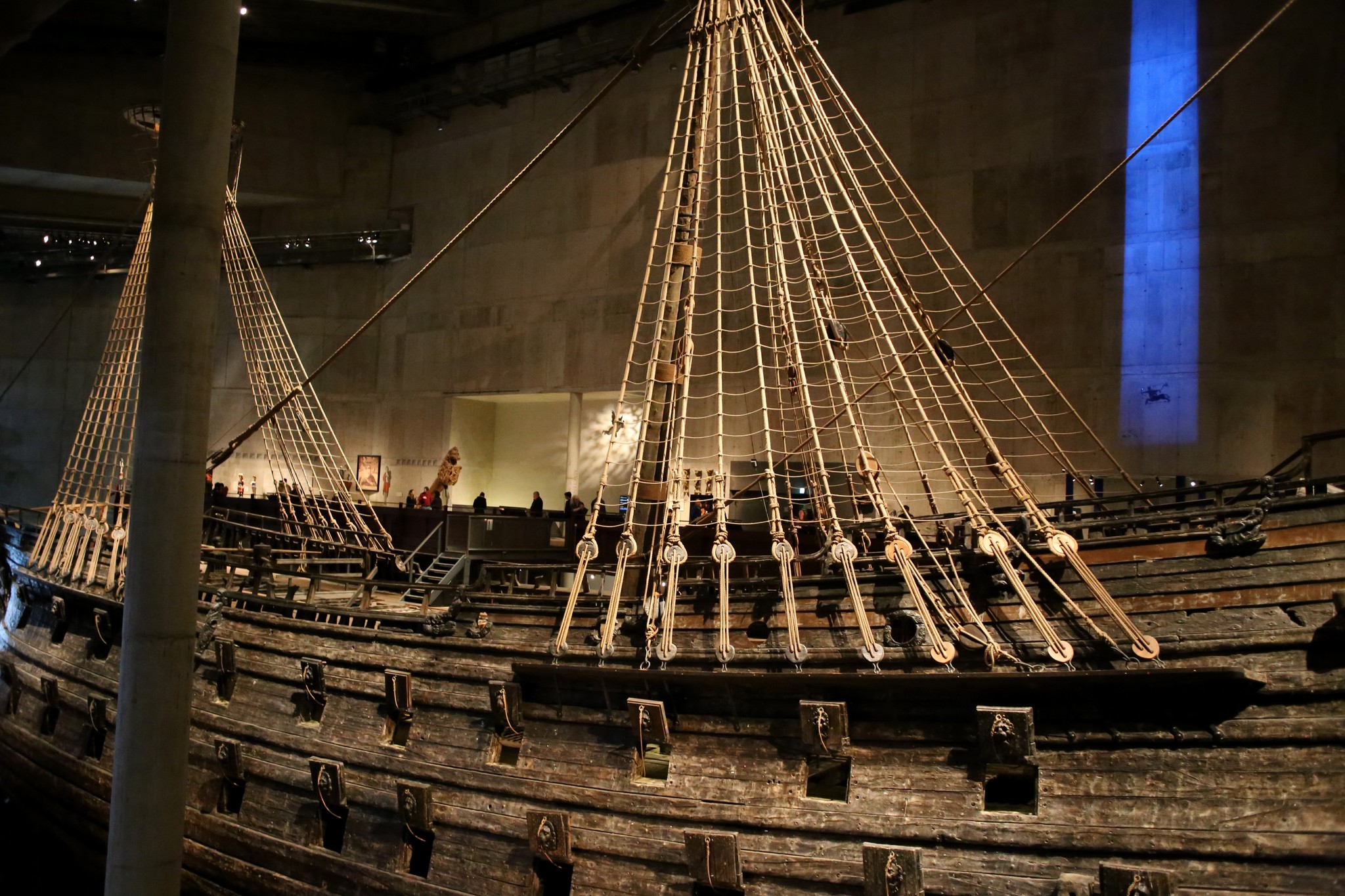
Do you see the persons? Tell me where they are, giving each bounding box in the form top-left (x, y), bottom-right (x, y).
top-left (237, 473), bottom-right (245, 498)
top-left (564, 492), bottom-right (572, 514)
top-left (406, 489), bottom-right (417, 509)
top-left (419, 486), bottom-right (433, 510)
top-left (666, 509), bottom-right (672, 523)
top-left (794, 510), bottom-right (809, 526)
top-left (591, 491), bottom-right (606, 515)
top-left (899, 505), bottom-right (914, 532)
top-left (691, 499), bottom-right (727, 528)
top-left (570, 495), bottom-right (586, 520)
top-left (204, 476), bottom-right (228, 497)
top-left (277, 478), bottom-right (302, 496)
top-left (430, 490), bottom-right (442, 511)
top-left (473, 492), bottom-right (487, 513)
top-left (250, 476), bottom-right (256, 495)
top-left (530, 491), bottom-right (543, 518)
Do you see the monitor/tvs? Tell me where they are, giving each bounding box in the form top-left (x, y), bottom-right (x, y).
top-left (619, 496), bottom-right (628, 510)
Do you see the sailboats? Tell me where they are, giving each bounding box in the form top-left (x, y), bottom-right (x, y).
top-left (0, 0), bottom-right (1345, 896)
